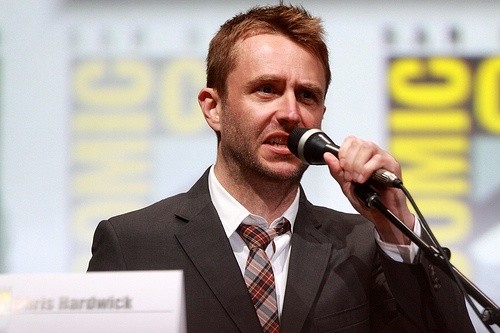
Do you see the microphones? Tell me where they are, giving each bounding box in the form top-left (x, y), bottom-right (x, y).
top-left (287, 128), bottom-right (402, 188)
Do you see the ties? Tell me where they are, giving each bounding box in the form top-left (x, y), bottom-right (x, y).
top-left (235, 217), bottom-right (291, 333)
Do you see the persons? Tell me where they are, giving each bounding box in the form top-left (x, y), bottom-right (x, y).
top-left (87, 3), bottom-right (476, 333)
